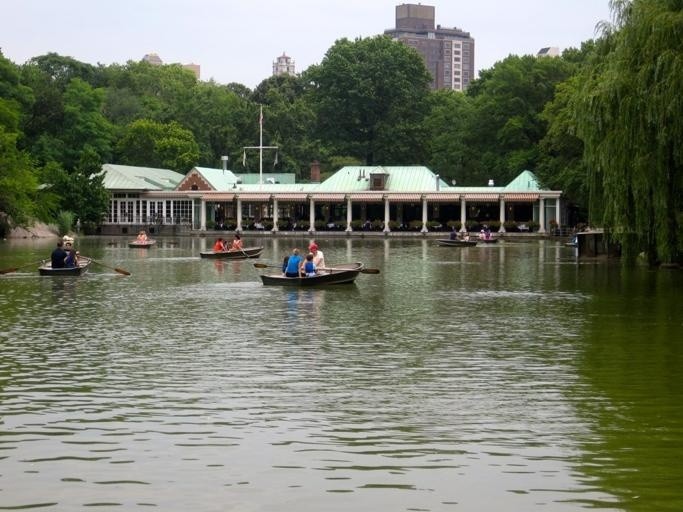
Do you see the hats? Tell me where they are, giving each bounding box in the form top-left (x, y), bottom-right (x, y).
top-left (308, 243), bottom-right (318, 252)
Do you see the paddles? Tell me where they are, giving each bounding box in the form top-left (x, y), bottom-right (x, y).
top-left (316, 268), bottom-right (380, 275)
top-left (239, 246), bottom-right (249, 259)
top-left (0, 256), bottom-right (52, 274)
top-left (254, 263), bottom-right (283, 268)
top-left (76, 255), bottom-right (131, 275)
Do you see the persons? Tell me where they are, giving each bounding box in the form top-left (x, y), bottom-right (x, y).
top-left (136, 230), bottom-right (147, 240)
top-left (50, 239), bottom-right (80, 269)
top-left (281, 243), bottom-right (325, 277)
top-left (214, 235), bottom-right (243, 253)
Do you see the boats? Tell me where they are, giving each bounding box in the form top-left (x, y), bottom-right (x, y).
top-left (260, 262), bottom-right (365, 287)
top-left (200, 247), bottom-right (264, 259)
top-left (38, 255), bottom-right (92, 276)
top-left (127, 239), bottom-right (156, 248)
top-left (436, 238), bottom-right (498, 248)
